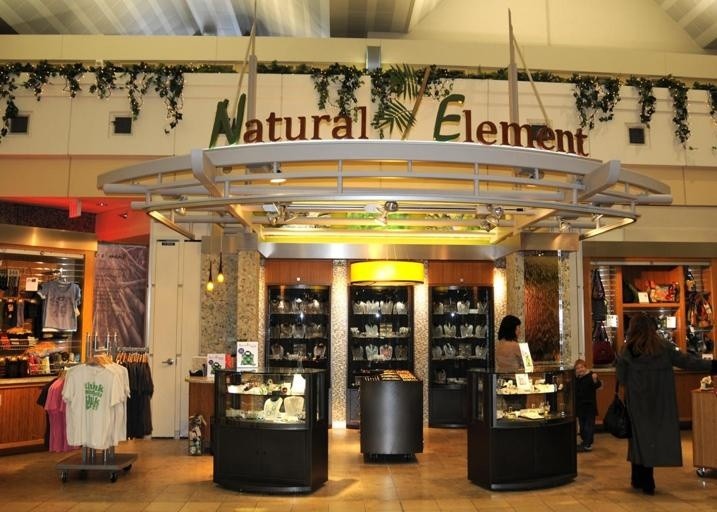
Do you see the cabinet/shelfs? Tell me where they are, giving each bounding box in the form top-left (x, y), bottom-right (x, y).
top-left (467, 364), bottom-right (582, 492)
top-left (0, 225), bottom-right (98, 458)
top-left (581, 242), bottom-right (717, 479)
top-left (211, 366), bottom-right (329, 496)
top-left (185, 376), bottom-right (215, 457)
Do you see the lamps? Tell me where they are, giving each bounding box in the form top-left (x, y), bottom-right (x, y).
top-left (373, 202), bottom-right (397, 227)
top-left (349, 260), bottom-right (425, 286)
top-left (206, 253), bottom-right (225, 290)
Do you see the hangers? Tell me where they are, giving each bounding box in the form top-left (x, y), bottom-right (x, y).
top-left (40, 268), bottom-right (81, 288)
top-left (58, 351), bottom-right (149, 380)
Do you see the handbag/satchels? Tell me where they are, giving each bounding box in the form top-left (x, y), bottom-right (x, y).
top-left (623, 266), bottom-right (711, 355)
top-left (592, 269), bottom-right (614, 364)
top-left (603, 379), bottom-right (630, 438)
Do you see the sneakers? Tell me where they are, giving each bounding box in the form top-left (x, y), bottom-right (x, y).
top-left (577, 442), bottom-right (593, 452)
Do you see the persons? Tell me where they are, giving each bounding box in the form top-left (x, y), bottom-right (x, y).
top-left (566, 358), bottom-right (602, 451)
top-left (496, 315), bottom-right (531, 412)
top-left (612, 312), bottom-right (716, 495)
